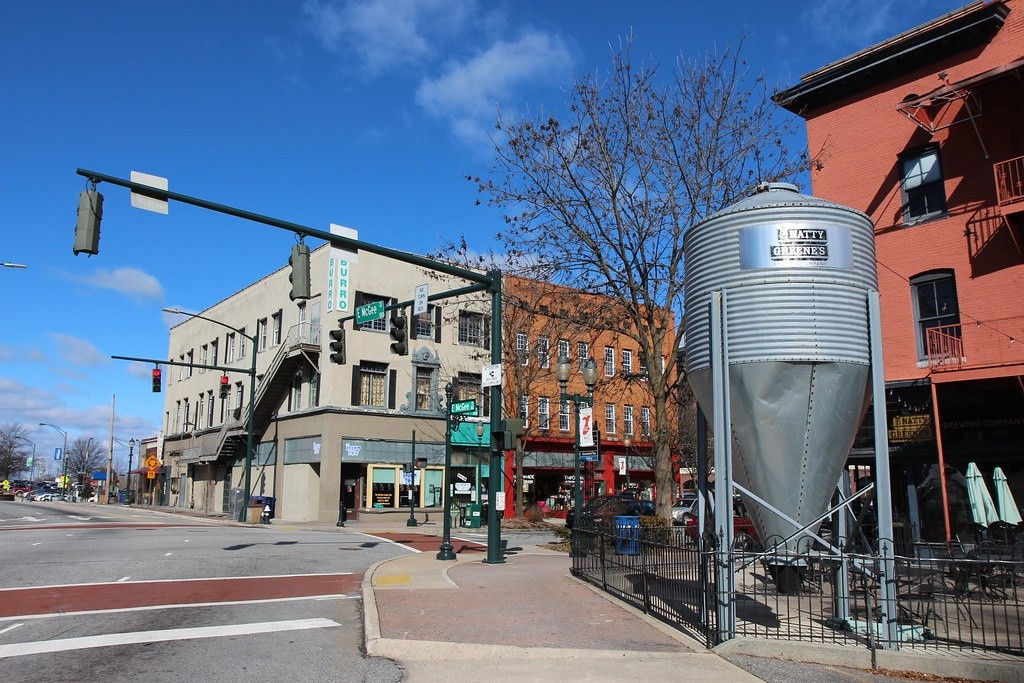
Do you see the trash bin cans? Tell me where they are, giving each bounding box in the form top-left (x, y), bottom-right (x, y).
top-left (118, 489), bottom-right (126, 503)
top-left (246, 499), bottom-right (265, 524)
top-left (464, 504), bottom-right (482, 528)
top-left (614, 516), bottom-right (641, 556)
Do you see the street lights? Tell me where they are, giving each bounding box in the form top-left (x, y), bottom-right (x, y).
top-left (39, 421), bottom-right (67, 501)
top-left (123, 437), bottom-right (135, 504)
top-left (475, 419), bottom-right (484, 524)
top-left (555, 350), bottom-right (599, 560)
top-left (15, 436), bottom-right (36, 500)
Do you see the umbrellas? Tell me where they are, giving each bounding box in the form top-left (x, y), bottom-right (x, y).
top-left (991, 467), bottom-right (1022, 545)
top-left (964, 461), bottom-right (1000, 542)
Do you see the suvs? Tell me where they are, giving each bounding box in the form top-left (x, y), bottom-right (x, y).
top-left (684, 495), bottom-right (766, 552)
top-left (566, 487), bottom-right (644, 529)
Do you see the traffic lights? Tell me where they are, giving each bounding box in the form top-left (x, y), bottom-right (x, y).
top-left (389, 317), bottom-right (406, 355)
top-left (220, 376), bottom-right (228, 399)
top-left (403, 463), bottom-right (407, 473)
top-left (416, 457), bottom-right (427, 467)
top-left (328, 330), bottom-right (343, 364)
top-left (287, 253), bottom-right (296, 302)
top-left (153, 369), bottom-right (161, 393)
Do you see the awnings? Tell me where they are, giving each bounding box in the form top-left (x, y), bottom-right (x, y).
top-left (128, 467), bottom-right (159, 474)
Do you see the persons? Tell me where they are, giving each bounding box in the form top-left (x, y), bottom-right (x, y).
top-left (337, 485), bottom-right (353, 526)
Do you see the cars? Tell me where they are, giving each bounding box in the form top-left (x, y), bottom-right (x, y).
top-left (582, 499), bottom-right (656, 526)
top-left (671, 496), bottom-right (702, 527)
top-left (0, 478), bottom-right (117, 503)
